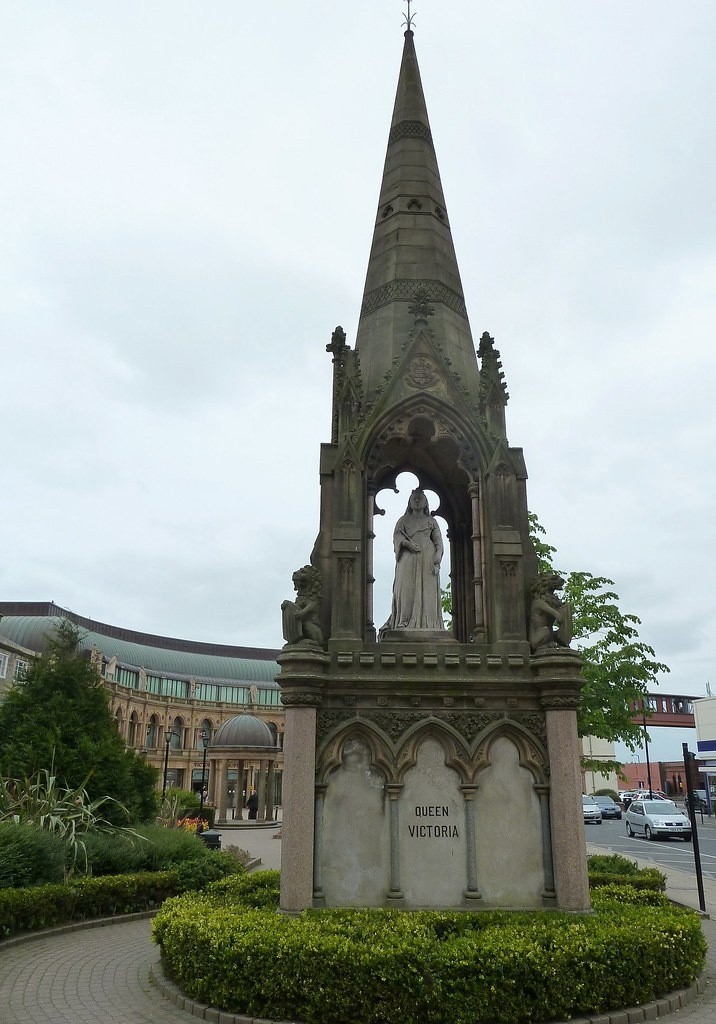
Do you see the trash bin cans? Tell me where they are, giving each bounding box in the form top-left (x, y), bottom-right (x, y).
top-left (200, 830), bottom-right (222, 850)
top-left (623, 798), bottom-right (632, 812)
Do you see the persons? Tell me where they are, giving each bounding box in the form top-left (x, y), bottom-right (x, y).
top-left (107, 654), bottom-right (117, 680)
top-left (246, 790), bottom-right (259, 820)
top-left (190, 674), bottom-right (196, 696)
top-left (139, 666), bottom-right (147, 690)
top-left (378, 490), bottom-right (448, 630)
top-left (248, 682), bottom-right (258, 702)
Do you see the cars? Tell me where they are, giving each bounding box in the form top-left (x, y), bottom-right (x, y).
top-left (590, 796), bottom-right (622, 820)
top-left (582, 795), bottom-right (602, 824)
top-left (624, 801), bottom-right (692, 842)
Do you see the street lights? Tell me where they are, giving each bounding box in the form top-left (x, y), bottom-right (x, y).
top-left (200, 733), bottom-right (209, 809)
top-left (162, 727), bottom-right (173, 805)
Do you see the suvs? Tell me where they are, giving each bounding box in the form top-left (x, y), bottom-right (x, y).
top-left (684, 790), bottom-right (708, 814)
top-left (618, 789), bottom-right (675, 807)
